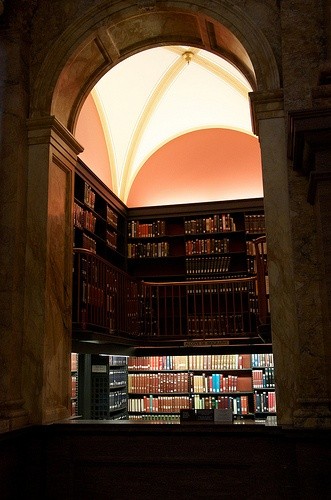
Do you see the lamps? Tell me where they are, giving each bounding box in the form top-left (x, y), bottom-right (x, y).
top-left (180, 51), bottom-right (194, 66)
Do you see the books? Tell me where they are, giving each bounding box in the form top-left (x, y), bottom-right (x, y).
top-left (66, 179), bottom-right (277, 421)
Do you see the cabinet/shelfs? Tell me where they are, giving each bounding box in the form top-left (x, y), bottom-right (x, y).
top-left (66, 171), bottom-right (283, 429)
